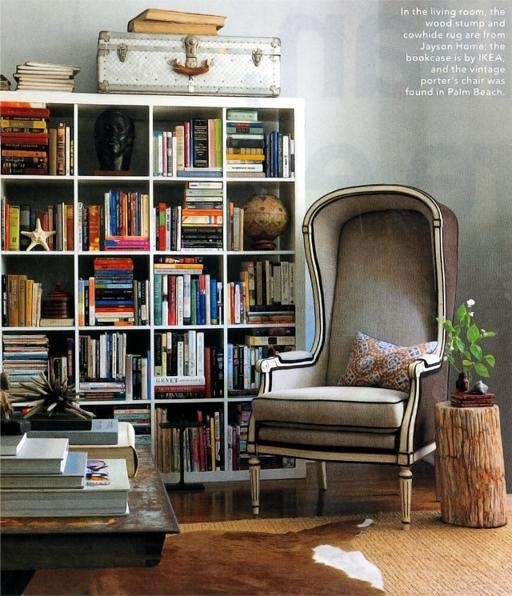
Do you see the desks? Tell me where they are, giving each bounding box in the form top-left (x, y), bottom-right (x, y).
top-left (2, 439), bottom-right (185, 570)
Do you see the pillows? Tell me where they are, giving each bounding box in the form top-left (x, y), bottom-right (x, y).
top-left (334, 331), bottom-right (443, 396)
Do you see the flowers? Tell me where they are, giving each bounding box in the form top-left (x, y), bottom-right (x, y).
top-left (437, 296), bottom-right (497, 372)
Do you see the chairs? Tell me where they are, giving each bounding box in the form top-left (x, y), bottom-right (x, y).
top-left (244, 181), bottom-right (461, 531)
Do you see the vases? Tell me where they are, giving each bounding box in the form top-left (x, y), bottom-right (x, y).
top-left (456, 372), bottom-right (469, 391)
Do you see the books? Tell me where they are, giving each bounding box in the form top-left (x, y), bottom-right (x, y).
top-left (132, 19), bottom-right (218, 35)
top-left (1, 457), bottom-right (132, 522)
top-left (1, 451), bottom-right (88, 490)
top-left (126, 7), bottom-right (227, 33)
top-left (0, 61), bottom-right (81, 92)
top-left (1, 99), bottom-right (295, 475)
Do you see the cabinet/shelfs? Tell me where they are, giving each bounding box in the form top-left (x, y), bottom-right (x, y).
top-left (0, 85), bottom-right (313, 488)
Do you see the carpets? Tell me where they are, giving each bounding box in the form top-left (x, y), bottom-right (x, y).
top-left (15, 517), bottom-right (393, 595)
